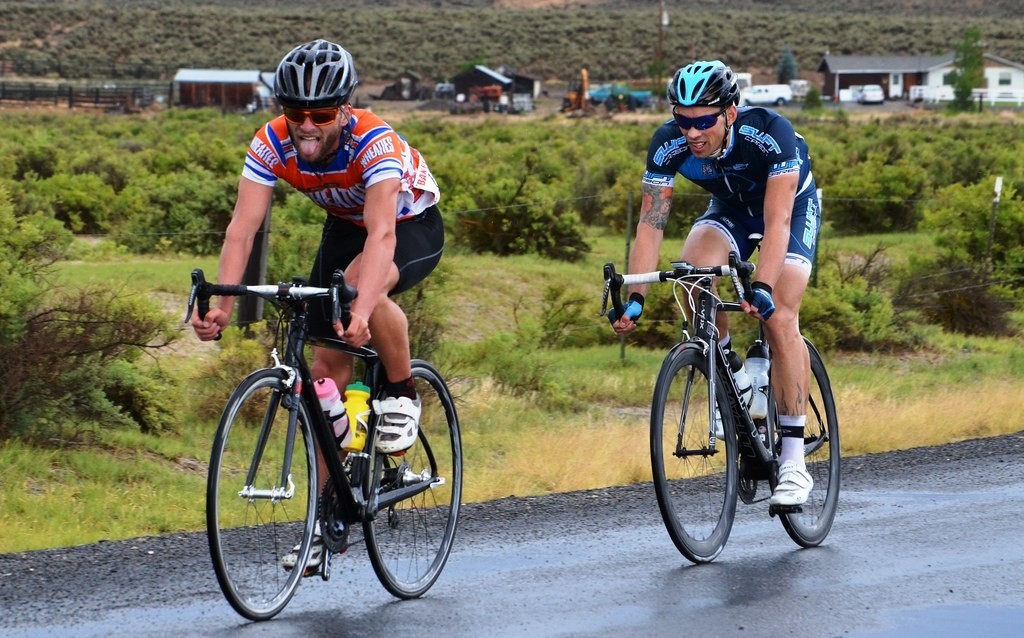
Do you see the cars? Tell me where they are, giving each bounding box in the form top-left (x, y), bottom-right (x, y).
top-left (857, 84), bottom-right (884, 105)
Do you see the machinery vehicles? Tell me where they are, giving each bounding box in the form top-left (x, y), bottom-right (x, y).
top-left (558, 69), bottom-right (596, 118)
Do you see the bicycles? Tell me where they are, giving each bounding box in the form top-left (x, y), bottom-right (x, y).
top-left (184, 268), bottom-right (463, 620)
top-left (600, 251), bottom-right (841, 565)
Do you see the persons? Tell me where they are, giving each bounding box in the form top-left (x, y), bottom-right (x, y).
top-left (609, 61), bottom-right (822, 506)
top-left (191, 38), bottom-right (445, 568)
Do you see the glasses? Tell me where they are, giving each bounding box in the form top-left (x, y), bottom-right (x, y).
top-left (672, 106), bottom-right (726, 130)
top-left (282, 105), bottom-right (338, 126)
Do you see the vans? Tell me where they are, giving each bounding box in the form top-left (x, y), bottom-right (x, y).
top-left (744, 85), bottom-right (792, 106)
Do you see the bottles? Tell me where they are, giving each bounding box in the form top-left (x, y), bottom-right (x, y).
top-left (314, 375), bottom-right (370, 453)
top-left (722, 340), bottom-right (770, 419)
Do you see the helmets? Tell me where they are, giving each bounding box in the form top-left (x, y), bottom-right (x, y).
top-left (668, 61), bottom-right (739, 109)
top-left (274, 39), bottom-right (358, 111)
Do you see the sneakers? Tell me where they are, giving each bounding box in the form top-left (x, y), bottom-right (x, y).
top-left (280, 517), bottom-right (325, 567)
top-left (771, 460), bottom-right (814, 505)
top-left (373, 393), bottom-right (422, 452)
top-left (715, 401), bottom-right (725, 439)
